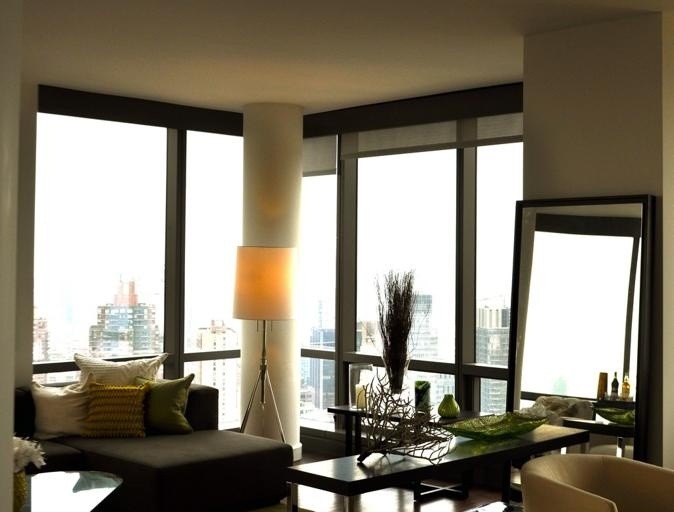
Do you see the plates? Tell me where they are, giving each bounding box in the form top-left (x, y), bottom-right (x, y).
top-left (442, 410), bottom-right (551, 441)
top-left (593, 407), bottom-right (635, 425)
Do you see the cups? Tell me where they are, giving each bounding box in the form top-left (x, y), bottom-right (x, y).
top-left (414, 380), bottom-right (430, 416)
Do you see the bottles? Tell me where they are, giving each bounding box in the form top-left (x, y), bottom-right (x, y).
top-left (597, 372), bottom-right (608, 400)
top-left (621, 372), bottom-right (630, 400)
top-left (610, 371), bottom-right (619, 399)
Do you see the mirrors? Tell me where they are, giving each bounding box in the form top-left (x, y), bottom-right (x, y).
top-left (501, 196), bottom-right (655, 502)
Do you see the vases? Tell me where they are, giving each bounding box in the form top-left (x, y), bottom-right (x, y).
top-left (438, 394), bottom-right (461, 420)
top-left (14, 471), bottom-right (31, 510)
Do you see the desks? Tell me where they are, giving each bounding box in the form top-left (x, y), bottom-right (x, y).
top-left (28, 470), bottom-right (124, 512)
top-left (559, 417), bottom-right (634, 460)
top-left (286, 406), bottom-right (591, 512)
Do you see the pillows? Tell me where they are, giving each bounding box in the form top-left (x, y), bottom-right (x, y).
top-left (31, 353), bottom-right (196, 440)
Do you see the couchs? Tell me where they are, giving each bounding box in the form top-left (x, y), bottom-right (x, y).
top-left (14, 384), bottom-right (295, 512)
top-left (523, 395), bottom-right (593, 428)
top-left (519, 450), bottom-right (674, 511)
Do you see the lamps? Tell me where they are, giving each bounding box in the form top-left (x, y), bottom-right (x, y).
top-left (236, 246), bottom-right (296, 443)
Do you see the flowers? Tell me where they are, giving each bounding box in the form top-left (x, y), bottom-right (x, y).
top-left (14, 434), bottom-right (47, 472)
